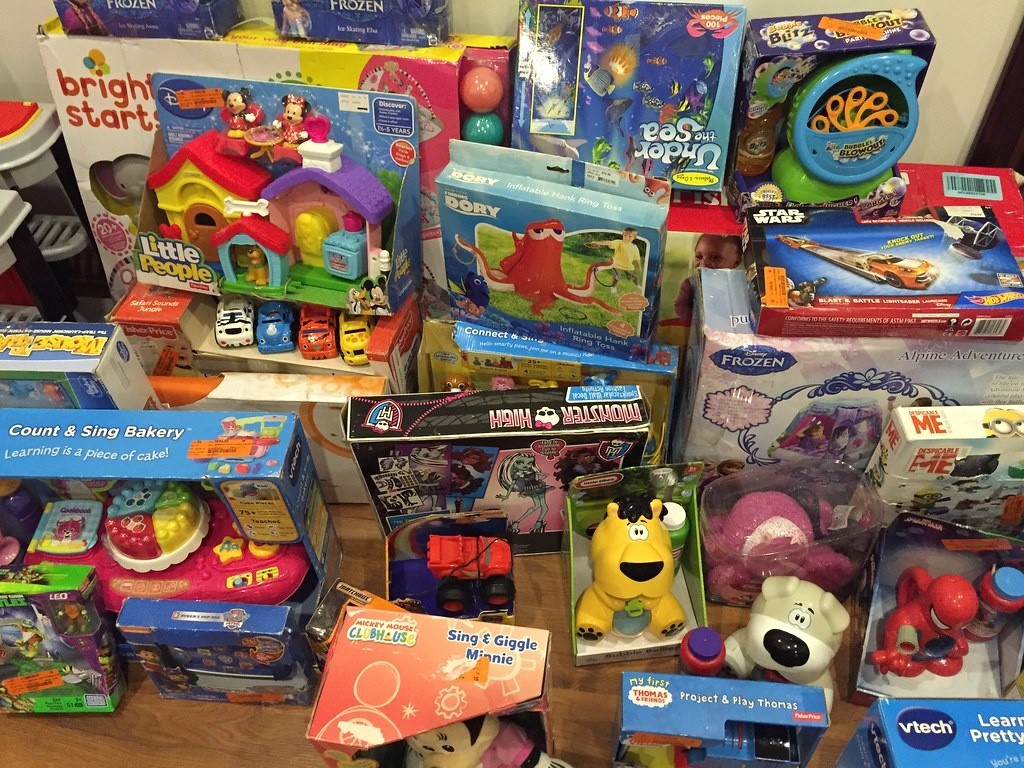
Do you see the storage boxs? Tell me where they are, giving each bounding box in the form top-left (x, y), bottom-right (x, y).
top-left (0, 321), bottom-right (163, 410)
top-left (146, 375), bottom-right (391, 504)
top-left (116, 596), bottom-right (318, 707)
top-left (339, 385), bottom-right (653, 557)
top-left (510, 0), bottom-right (746, 192)
top-left (850, 405), bottom-right (1024, 533)
top-left (271, 0), bottom-right (449, 46)
top-left (305, 605), bottom-right (556, 768)
top-left (37, 18), bottom-right (517, 302)
top-left (833, 697), bottom-right (1024, 768)
top-left (435, 139), bottom-right (670, 366)
top-left (728, 5), bottom-right (936, 216)
top-left (52, 0), bottom-right (246, 41)
top-left (0, 408), bottom-right (342, 588)
top-left (898, 162), bottom-right (1024, 265)
top-left (105, 278), bottom-right (422, 395)
top-left (675, 267), bottom-right (1024, 474)
top-left (743, 206), bottom-right (1024, 341)
top-left (611, 672), bottom-right (832, 768)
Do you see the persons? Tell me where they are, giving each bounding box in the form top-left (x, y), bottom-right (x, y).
top-left (589, 226), bottom-right (644, 293)
top-left (673, 234), bottom-right (745, 321)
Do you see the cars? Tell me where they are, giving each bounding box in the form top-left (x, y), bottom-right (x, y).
top-left (216, 295), bottom-right (255, 349)
top-left (255, 302), bottom-right (296, 354)
top-left (299, 305), bottom-right (338, 361)
top-left (337, 309), bottom-right (371, 367)
